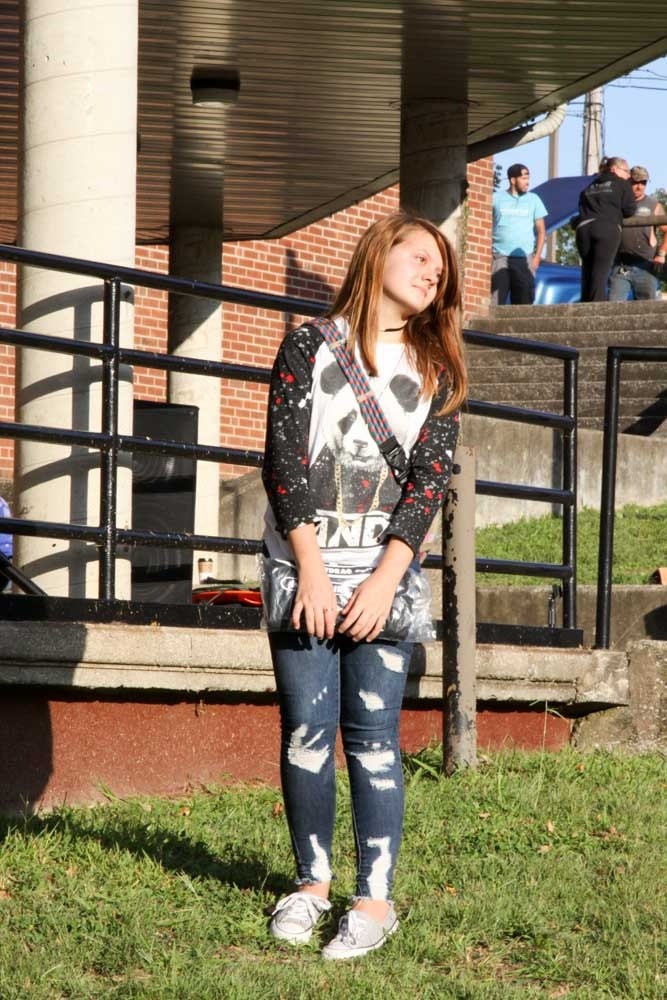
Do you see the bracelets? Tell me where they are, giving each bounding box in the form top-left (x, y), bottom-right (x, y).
top-left (657, 251), bottom-right (666, 257)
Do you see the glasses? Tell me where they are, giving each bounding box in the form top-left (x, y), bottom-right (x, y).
top-left (619, 168), bottom-right (631, 173)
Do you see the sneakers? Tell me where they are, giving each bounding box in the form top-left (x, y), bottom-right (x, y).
top-left (322, 906), bottom-right (399, 960)
top-left (270, 893), bottom-right (333, 945)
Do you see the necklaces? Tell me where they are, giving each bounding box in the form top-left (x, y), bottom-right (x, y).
top-left (377, 318), bottom-right (408, 332)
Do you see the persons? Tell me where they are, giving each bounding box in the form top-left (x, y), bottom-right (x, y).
top-left (608, 165), bottom-right (667, 302)
top-left (490, 163), bottom-right (550, 306)
top-left (255, 215), bottom-right (470, 960)
top-left (575, 156), bottom-right (637, 302)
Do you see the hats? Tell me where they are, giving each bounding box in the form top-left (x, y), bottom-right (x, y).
top-left (506, 164), bottom-right (529, 178)
top-left (631, 166), bottom-right (649, 182)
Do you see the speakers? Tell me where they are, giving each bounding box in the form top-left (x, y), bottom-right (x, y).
top-left (132, 398), bottom-right (199, 606)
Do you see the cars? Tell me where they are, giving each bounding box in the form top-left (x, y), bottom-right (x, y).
top-left (503, 173), bottom-right (661, 305)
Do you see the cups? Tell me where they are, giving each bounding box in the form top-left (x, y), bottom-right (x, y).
top-left (198, 556), bottom-right (213, 584)
